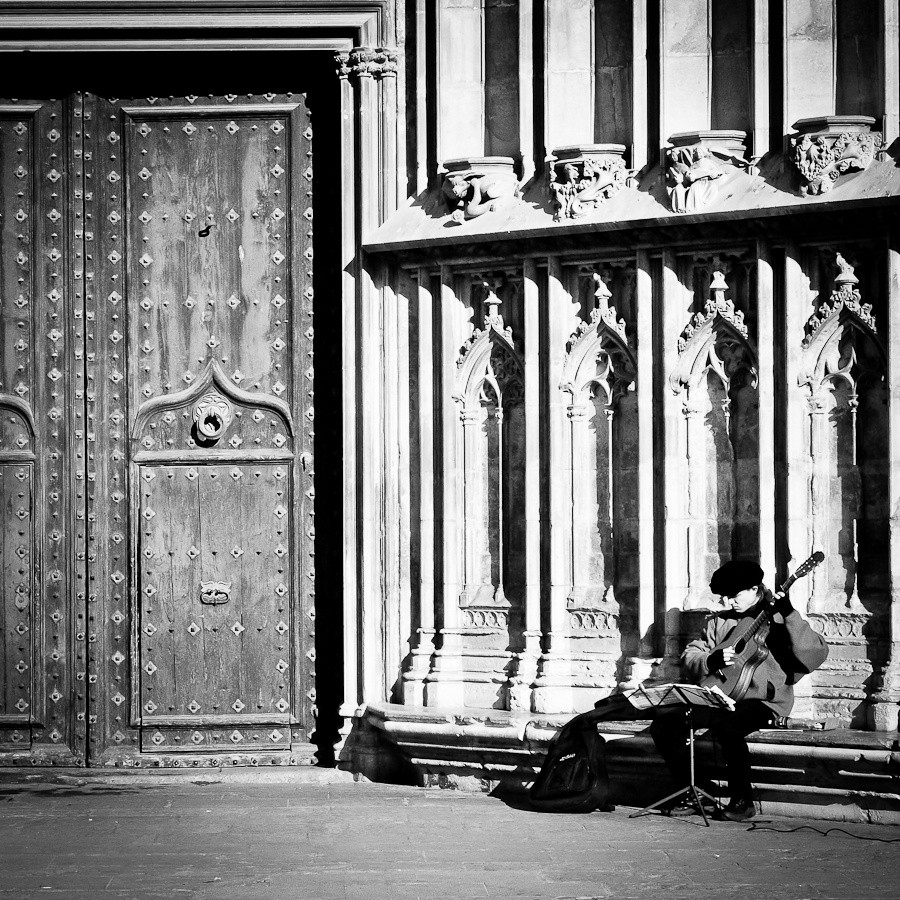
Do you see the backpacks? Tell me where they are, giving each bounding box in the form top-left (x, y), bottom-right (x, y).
top-left (528, 715), bottom-right (615, 811)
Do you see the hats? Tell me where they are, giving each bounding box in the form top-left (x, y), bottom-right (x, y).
top-left (709, 558), bottom-right (764, 598)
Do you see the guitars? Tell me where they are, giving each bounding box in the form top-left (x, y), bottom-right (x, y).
top-left (698, 551), bottom-right (825, 704)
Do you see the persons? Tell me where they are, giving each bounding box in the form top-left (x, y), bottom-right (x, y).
top-left (661, 557), bottom-right (830, 821)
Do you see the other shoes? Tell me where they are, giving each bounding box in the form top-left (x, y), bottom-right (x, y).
top-left (662, 790), bottom-right (714, 817)
top-left (718, 797), bottom-right (756, 821)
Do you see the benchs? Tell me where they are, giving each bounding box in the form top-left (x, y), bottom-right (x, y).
top-left (366, 702), bottom-right (899, 815)
